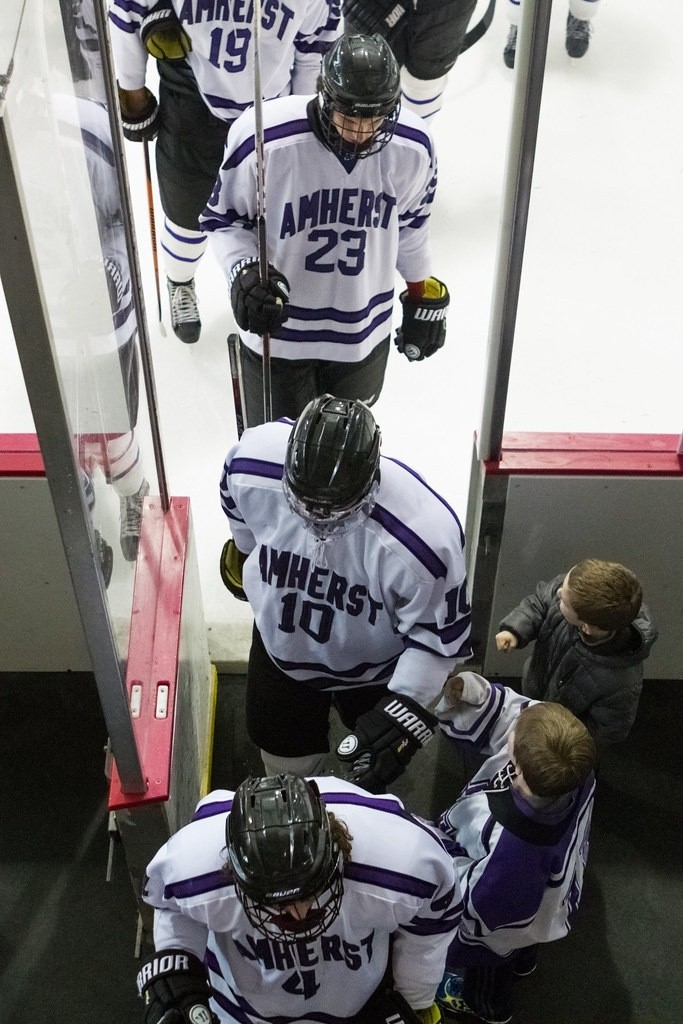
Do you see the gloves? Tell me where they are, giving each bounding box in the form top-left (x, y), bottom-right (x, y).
top-left (219, 538), bottom-right (249, 601)
top-left (334, 691), bottom-right (439, 798)
top-left (103, 257), bottom-right (124, 314)
top-left (340, 0), bottom-right (414, 41)
top-left (115, 80), bottom-right (162, 141)
top-left (140, 0), bottom-right (194, 60)
top-left (136, 949), bottom-right (220, 1024)
top-left (230, 260), bottom-right (290, 338)
top-left (395, 277), bottom-right (450, 362)
top-left (381, 992), bottom-right (442, 1024)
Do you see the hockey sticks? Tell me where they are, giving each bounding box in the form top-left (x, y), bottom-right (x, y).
top-left (248, 0), bottom-right (284, 423)
top-left (463, 0), bottom-right (499, 51)
top-left (139, 138), bottom-right (167, 338)
top-left (227, 332), bottom-right (251, 439)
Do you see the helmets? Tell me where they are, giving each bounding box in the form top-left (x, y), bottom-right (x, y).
top-left (317, 30), bottom-right (404, 160)
top-left (282, 394), bottom-right (381, 541)
top-left (224, 775), bottom-right (342, 945)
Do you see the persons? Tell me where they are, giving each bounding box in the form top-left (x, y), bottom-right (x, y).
top-left (220, 394), bottom-right (472, 795)
top-left (502, 0), bottom-right (600, 71)
top-left (106, 0), bottom-right (346, 344)
top-left (434, 672), bottom-right (597, 1024)
top-left (51, 1), bottom-right (150, 562)
top-left (497, 559), bottom-right (659, 779)
top-left (342, 0), bottom-right (497, 126)
top-left (200, 35), bottom-right (447, 429)
top-left (136, 775), bottom-right (463, 1024)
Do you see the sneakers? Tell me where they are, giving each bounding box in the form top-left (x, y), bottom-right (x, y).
top-left (434, 972), bottom-right (512, 1024)
top-left (166, 277), bottom-right (202, 355)
top-left (504, 23), bottom-right (519, 70)
top-left (507, 958), bottom-right (538, 976)
top-left (119, 479), bottom-right (150, 565)
top-left (565, 10), bottom-right (590, 64)
